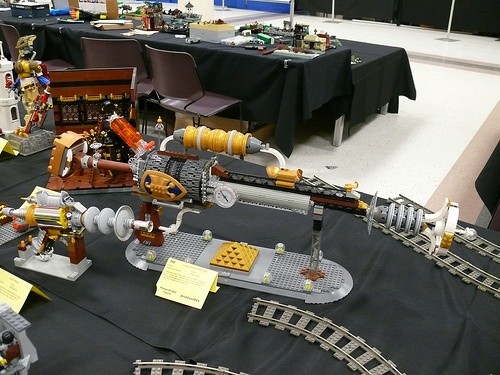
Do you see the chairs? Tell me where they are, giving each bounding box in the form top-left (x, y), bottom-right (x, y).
top-left (80, 36), bottom-right (157, 134)
top-left (144, 42), bottom-right (245, 132)
top-left (0, 23), bottom-right (75, 74)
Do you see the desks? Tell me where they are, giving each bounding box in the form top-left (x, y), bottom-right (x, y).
top-left (0, 141), bottom-right (500, 375)
top-left (0, 7), bottom-right (417, 147)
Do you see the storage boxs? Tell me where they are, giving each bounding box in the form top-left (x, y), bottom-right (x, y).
top-left (190, 21), bottom-right (236, 44)
top-left (9, 2), bottom-right (50, 18)
